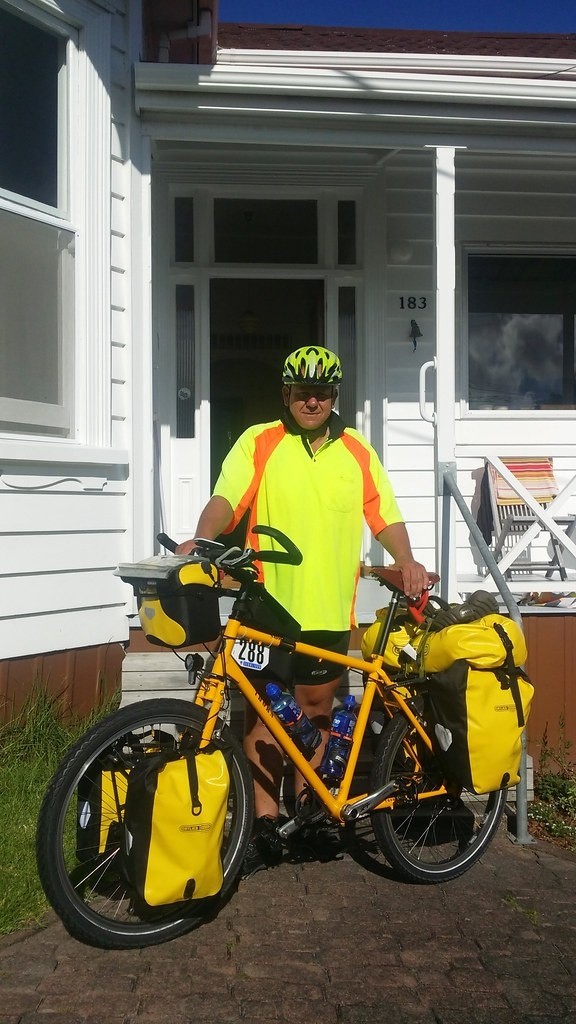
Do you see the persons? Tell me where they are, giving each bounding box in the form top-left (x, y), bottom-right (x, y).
top-left (175, 345), bottom-right (429, 880)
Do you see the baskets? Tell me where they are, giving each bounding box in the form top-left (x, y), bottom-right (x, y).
top-left (113, 554), bottom-right (223, 650)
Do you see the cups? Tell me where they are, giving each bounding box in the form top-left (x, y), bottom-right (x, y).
top-left (458, 592), bottom-right (471, 603)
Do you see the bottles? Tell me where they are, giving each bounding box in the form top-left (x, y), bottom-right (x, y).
top-left (265, 683), bottom-right (322, 749)
top-left (319, 695), bottom-right (357, 779)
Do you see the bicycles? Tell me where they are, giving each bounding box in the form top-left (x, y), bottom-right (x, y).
top-left (37, 526), bottom-right (508, 949)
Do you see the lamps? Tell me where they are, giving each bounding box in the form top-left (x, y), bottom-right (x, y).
top-left (409, 320), bottom-right (423, 355)
top-left (238, 211), bottom-right (260, 335)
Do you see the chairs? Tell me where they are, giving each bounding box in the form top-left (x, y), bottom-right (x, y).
top-left (483, 455), bottom-right (575, 584)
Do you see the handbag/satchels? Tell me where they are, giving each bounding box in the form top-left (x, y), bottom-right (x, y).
top-left (74, 728), bottom-right (232, 916)
top-left (360, 591), bottom-right (537, 796)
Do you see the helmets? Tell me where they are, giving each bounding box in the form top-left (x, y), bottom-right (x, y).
top-left (281, 346), bottom-right (342, 386)
